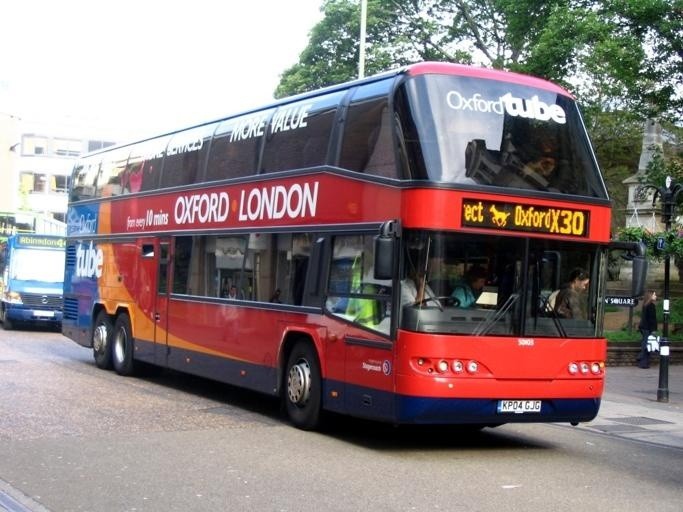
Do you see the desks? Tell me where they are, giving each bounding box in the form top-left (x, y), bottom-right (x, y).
top-left (636, 175), bottom-right (682, 403)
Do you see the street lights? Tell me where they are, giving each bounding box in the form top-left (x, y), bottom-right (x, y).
top-left (545, 289), bottom-right (561, 312)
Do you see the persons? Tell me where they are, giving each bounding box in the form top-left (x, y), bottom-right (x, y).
top-left (638, 289), bottom-right (657, 369)
top-left (400, 255), bottom-right (488, 307)
top-left (558, 267), bottom-right (591, 320)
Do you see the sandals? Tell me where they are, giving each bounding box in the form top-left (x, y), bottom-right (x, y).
top-left (0, 231), bottom-right (66, 330)
top-left (61, 62), bottom-right (651, 434)
